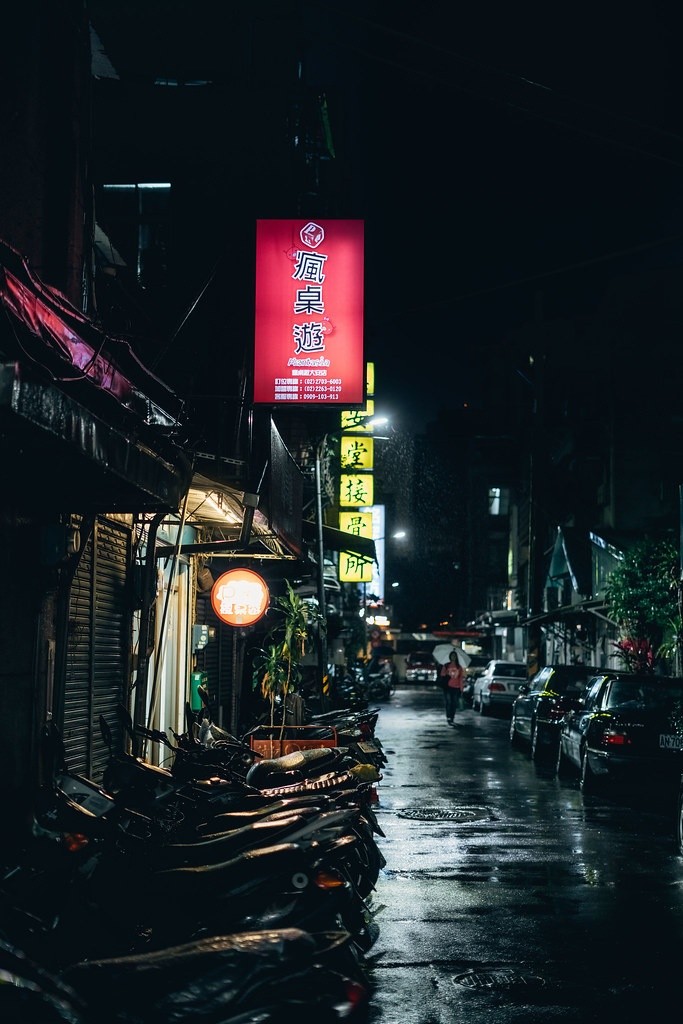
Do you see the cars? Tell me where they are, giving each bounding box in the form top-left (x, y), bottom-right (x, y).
top-left (461, 655), bottom-right (492, 707)
top-left (510, 663), bottom-right (605, 762)
top-left (404, 650), bottom-right (439, 684)
top-left (472, 658), bottom-right (528, 717)
top-left (553, 671), bottom-right (683, 798)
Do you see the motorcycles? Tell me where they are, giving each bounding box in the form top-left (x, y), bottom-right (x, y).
top-left (0, 653), bottom-right (395, 1024)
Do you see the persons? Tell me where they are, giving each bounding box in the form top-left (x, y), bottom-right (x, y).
top-left (527, 646), bottom-right (538, 676)
top-left (440, 651), bottom-right (463, 724)
top-left (190, 665), bottom-right (204, 725)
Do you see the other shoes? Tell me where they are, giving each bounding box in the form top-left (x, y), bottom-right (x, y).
top-left (447, 717), bottom-right (453, 724)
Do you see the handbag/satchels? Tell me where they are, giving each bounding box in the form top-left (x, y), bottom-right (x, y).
top-left (436, 664), bottom-right (451, 689)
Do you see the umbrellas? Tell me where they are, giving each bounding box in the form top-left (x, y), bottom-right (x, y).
top-left (432, 644), bottom-right (472, 668)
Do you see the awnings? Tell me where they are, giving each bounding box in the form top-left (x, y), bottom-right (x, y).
top-left (302, 520), bottom-right (376, 563)
